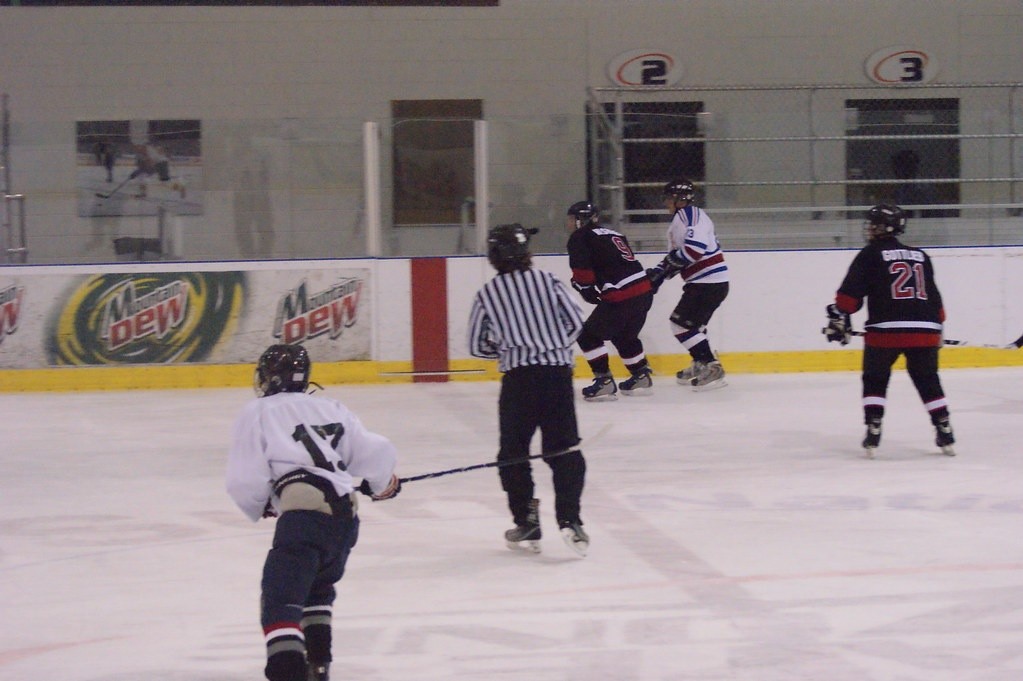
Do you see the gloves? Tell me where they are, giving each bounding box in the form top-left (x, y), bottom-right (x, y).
top-left (827, 304), bottom-right (851, 345)
top-left (663, 249), bottom-right (688, 280)
top-left (570, 278), bottom-right (600, 304)
top-left (263, 497), bottom-right (279, 519)
top-left (646, 268), bottom-right (665, 287)
top-left (361, 473), bottom-right (401, 502)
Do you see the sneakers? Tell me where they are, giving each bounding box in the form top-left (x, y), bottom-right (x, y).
top-left (862, 418), bottom-right (881, 459)
top-left (582, 376), bottom-right (618, 401)
top-left (676, 360), bottom-right (706, 384)
top-left (505, 499), bottom-right (541, 553)
top-left (306, 659), bottom-right (330, 681)
top-left (558, 498), bottom-right (590, 557)
top-left (936, 416), bottom-right (956, 456)
top-left (690, 359), bottom-right (728, 392)
top-left (619, 367), bottom-right (655, 394)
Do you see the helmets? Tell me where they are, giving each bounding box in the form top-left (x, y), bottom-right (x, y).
top-left (254, 344), bottom-right (311, 383)
top-left (862, 203), bottom-right (906, 242)
top-left (488, 223), bottom-right (531, 271)
top-left (663, 181), bottom-right (695, 196)
top-left (566, 201), bottom-right (600, 230)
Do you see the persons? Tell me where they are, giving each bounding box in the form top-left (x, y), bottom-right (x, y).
top-left (91, 138), bottom-right (112, 166)
top-left (649, 177), bottom-right (731, 391)
top-left (132, 143), bottom-right (185, 197)
top-left (566, 201), bottom-right (654, 403)
top-left (225, 343), bottom-right (401, 681)
top-left (466, 223), bottom-right (589, 556)
top-left (103, 150), bottom-right (115, 181)
top-left (848, 150), bottom-right (959, 218)
top-left (826, 203), bottom-right (957, 460)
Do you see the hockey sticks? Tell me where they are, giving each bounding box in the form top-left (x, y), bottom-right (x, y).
top-left (822, 325), bottom-right (1023, 350)
top-left (95, 176), bottom-right (135, 199)
top-left (352, 420), bottom-right (626, 493)
